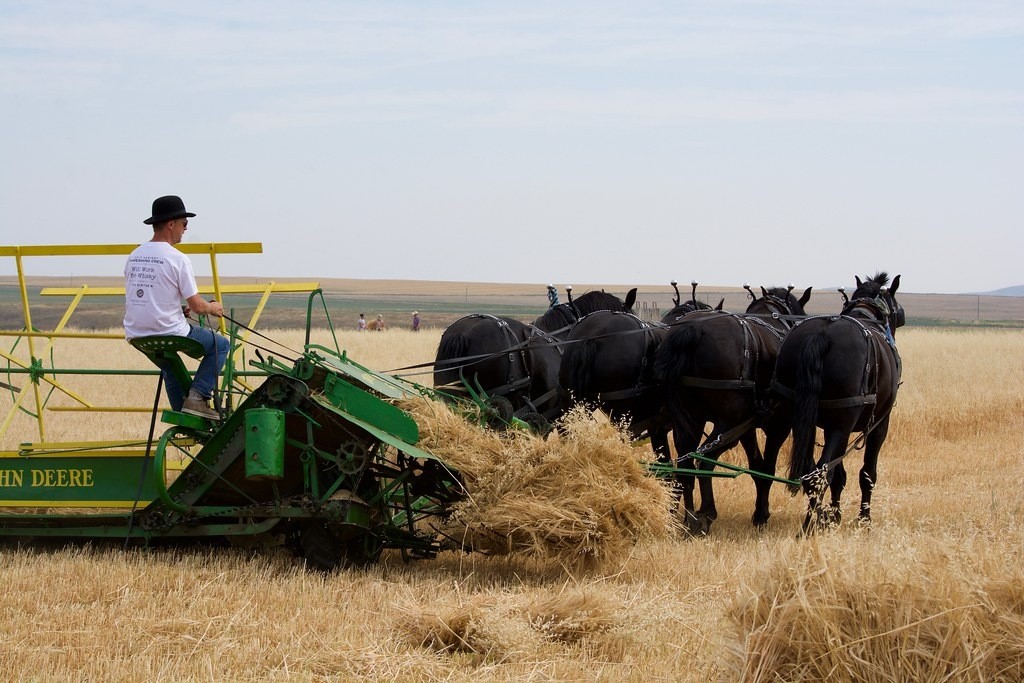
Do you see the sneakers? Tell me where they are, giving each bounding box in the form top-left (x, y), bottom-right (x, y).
top-left (181, 389), bottom-right (220, 420)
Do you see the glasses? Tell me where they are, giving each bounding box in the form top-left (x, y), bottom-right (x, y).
top-left (173, 219), bottom-right (188, 226)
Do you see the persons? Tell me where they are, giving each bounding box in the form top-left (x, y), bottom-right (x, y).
top-left (357, 313), bottom-right (385, 331)
top-left (121, 194), bottom-right (231, 420)
top-left (411, 310), bottom-right (421, 332)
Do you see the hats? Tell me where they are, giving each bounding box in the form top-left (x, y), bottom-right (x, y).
top-left (412, 311), bottom-right (418, 315)
top-left (378, 315), bottom-right (382, 318)
top-left (143, 195), bottom-right (196, 224)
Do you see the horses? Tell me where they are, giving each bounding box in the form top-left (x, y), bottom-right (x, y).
top-left (433, 271), bottom-right (906, 540)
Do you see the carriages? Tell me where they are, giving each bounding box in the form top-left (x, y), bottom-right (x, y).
top-left (0, 244), bottom-right (907, 569)
